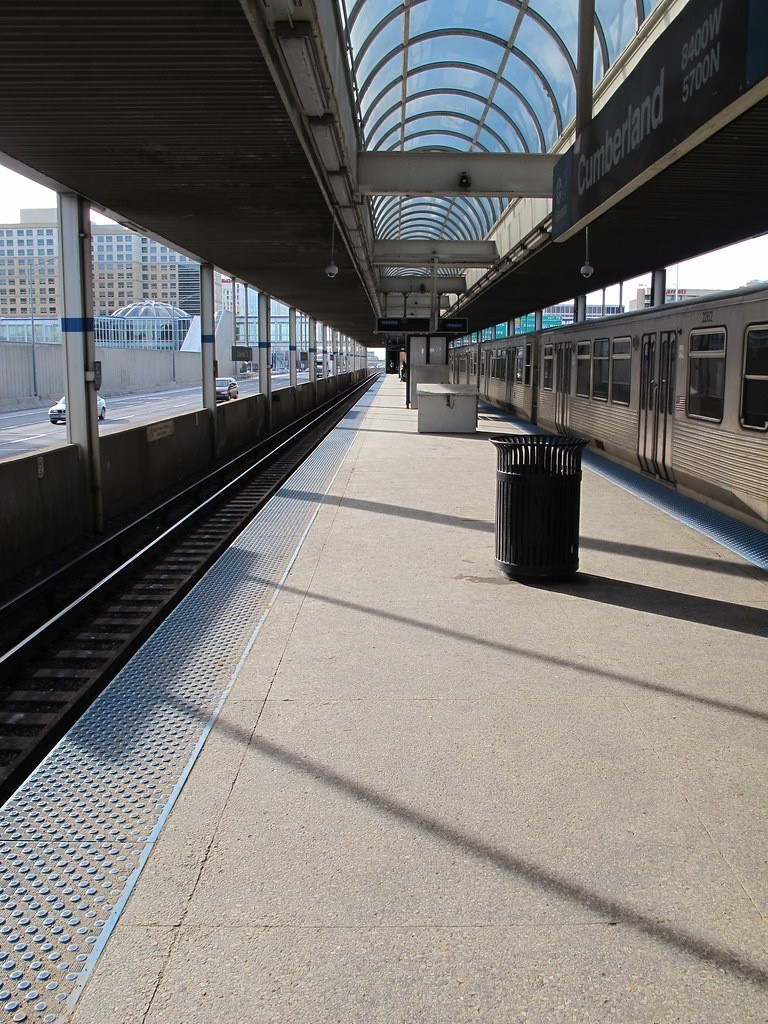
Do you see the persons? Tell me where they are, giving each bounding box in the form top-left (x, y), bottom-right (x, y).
top-left (389, 359), bottom-right (399, 374)
top-left (399, 361), bottom-right (406, 382)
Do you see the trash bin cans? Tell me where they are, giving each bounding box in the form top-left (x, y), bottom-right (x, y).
top-left (488, 434), bottom-right (591, 582)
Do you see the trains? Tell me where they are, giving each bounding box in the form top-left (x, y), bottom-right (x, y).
top-left (450, 279), bottom-right (768, 533)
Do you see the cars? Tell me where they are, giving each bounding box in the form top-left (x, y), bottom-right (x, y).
top-left (215, 377), bottom-right (238, 401)
top-left (305, 368), bottom-right (310, 372)
top-left (48, 393), bottom-right (106, 425)
top-left (317, 372), bottom-right (329, 378)
